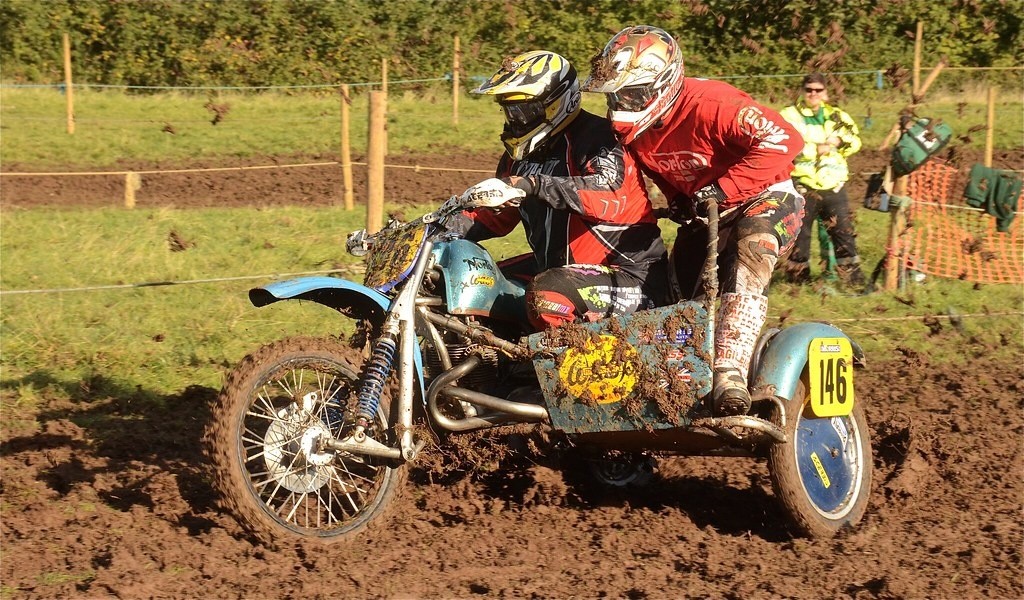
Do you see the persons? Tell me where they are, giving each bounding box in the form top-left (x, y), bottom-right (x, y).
top-left (387, 50), bottom-right (676, 344)
top-left (576, 24), bottom-right (808, 419)
top-left (777, 72), bottom-right (880, 293)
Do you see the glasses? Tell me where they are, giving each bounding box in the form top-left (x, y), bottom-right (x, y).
top-left (803, 87), bottom-right (826, 93)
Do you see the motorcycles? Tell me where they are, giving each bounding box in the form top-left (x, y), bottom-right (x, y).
top-left (206, 177), bottom-right (875, 550)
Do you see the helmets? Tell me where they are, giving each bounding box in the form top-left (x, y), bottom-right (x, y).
top-left (578, 24), bottom-right (685, 147)
top-left (469, 50), bottom-right (583, 163)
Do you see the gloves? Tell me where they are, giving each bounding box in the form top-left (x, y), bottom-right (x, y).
top-left (691, 182), bottom-right (727, 218)
top-left (486, 175), bottom-right (535, 216)
top-left (664, 192), bottom-right (697, 224)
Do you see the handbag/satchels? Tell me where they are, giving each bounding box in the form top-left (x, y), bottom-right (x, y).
top-left (863, 165), bottom-right (895, 213)
top-left (891, 115), bottom-right (953, 178)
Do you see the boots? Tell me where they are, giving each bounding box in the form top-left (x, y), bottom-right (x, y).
top-left (709, 292), bottom-right (769, 415)
